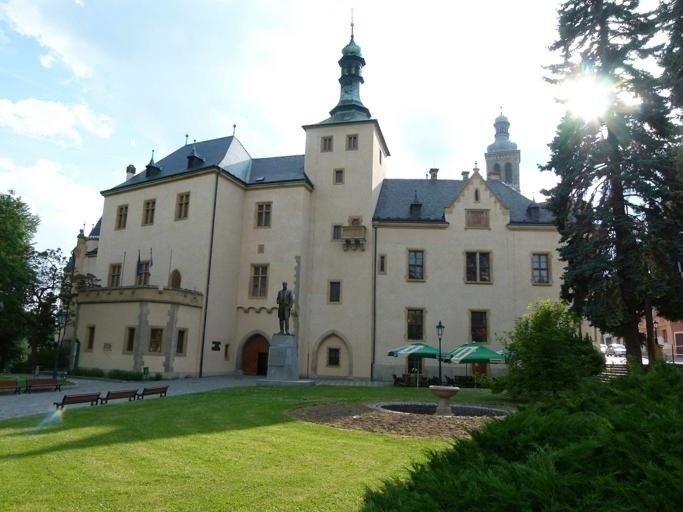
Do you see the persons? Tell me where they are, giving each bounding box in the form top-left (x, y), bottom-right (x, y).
top-left (276, 280), bottom-right (293, 335)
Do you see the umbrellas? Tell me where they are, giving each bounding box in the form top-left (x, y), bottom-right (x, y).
top-left (442, 341), bottom-right (506, 388)
top-left (387, 342), bottom-right (450, 386)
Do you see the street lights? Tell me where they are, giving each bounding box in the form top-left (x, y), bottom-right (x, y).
top-left (653, 320), bottom-right (658, 345)
top-left (434, 318), bottom-right (445, 387)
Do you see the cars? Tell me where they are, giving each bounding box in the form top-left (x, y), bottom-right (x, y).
top-left (606, 344), bottom-right (627, 357)
top-left (599, 343), bottom-right (608, 356)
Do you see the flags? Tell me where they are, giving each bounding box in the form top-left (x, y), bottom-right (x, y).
top-left (136, 251), bottom-right (142, 276)
top-left (147, 250), bottom-right (153, 276)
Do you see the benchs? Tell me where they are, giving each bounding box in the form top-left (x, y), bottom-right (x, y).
top-left (25, 379), bottom-right (63, 394)
top-left (53, 385), bottom-right (169, 410)
top-left (0, 379), bottom-right (21, 394)
